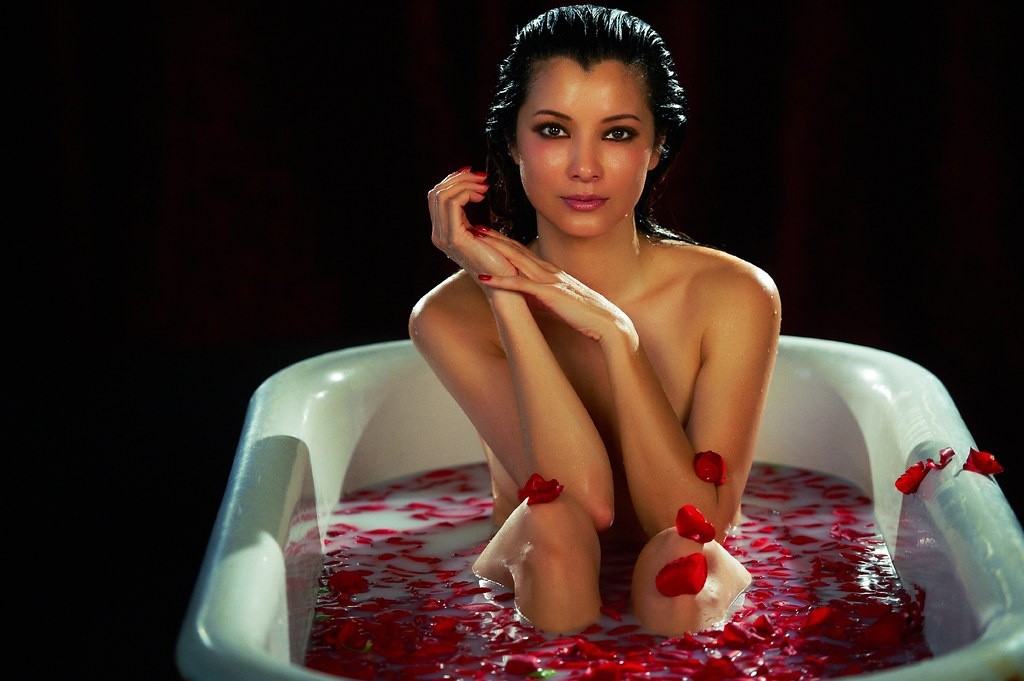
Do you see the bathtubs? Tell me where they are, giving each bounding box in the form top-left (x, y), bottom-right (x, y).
top-left (169, 327), bottom-right (1024, 681)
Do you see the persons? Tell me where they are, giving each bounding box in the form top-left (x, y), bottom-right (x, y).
top-left (411, 4), bottom-right (781, 634)
